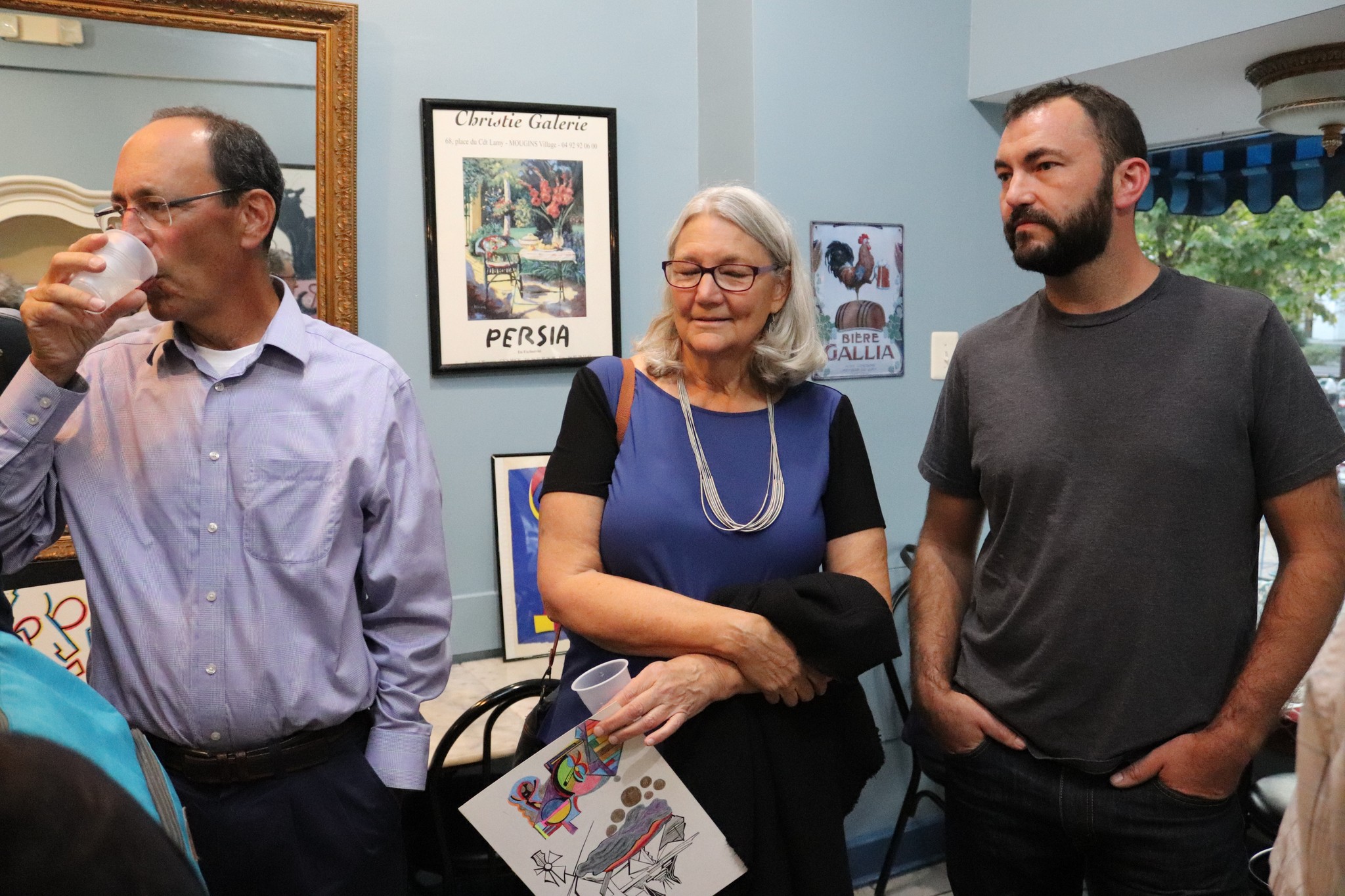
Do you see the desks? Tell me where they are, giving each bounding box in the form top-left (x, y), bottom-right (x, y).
top-left (419, 654), bottom-right (565, 769)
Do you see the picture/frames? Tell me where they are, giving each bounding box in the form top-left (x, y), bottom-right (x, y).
top-left (491, 452), bottom-right (570, 663)
top-left (422, 99), bottom-right (622, 379)
top-left (269, 163), bottom-right (318, 319)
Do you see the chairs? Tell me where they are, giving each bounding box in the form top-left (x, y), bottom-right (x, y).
top-left (429, 679), bottom-right (560, 896)
top-left (874, 580), bottom-right (945, 896)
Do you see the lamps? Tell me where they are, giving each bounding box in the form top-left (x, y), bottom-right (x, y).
top-left (1244, 42), bottom-right (1345, 157)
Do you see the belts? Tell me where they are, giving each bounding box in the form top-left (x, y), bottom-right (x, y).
top-left (144, 705), bottom-right (376, 784)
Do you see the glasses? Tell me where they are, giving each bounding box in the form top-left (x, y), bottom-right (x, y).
top-left (277, 274), bottom-right (298, 279)
top-left (662, 260), bottom-right (781, 293)
top-left (94, 187), bottom-right (233, 234)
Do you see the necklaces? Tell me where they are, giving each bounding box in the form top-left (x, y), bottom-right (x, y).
top-left (680, 373), bottom-right (785, 533)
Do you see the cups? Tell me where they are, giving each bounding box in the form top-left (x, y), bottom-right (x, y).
top-left (571, 658), bottom-right (632, 716)
top-left (68, 229), bottom-right (158, 315)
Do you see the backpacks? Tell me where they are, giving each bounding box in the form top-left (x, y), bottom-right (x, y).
top-left (1, 629), bottom-right (212, 896)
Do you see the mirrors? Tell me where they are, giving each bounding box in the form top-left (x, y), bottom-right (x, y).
top-left (0, 0), bottom-right (357, 560)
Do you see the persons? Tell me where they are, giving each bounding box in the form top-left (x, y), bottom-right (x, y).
top-left (0, 729), bottom-right (204, 896)
top-left (538, 184), bottom-right (894, 896)
top-left (0, 107), bottom-right (451, 896)
top-left (910, 78), bottom-right (1345, 896)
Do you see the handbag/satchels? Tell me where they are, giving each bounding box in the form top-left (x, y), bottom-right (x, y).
top-left (511, 685), bottom-right (561, 773)
